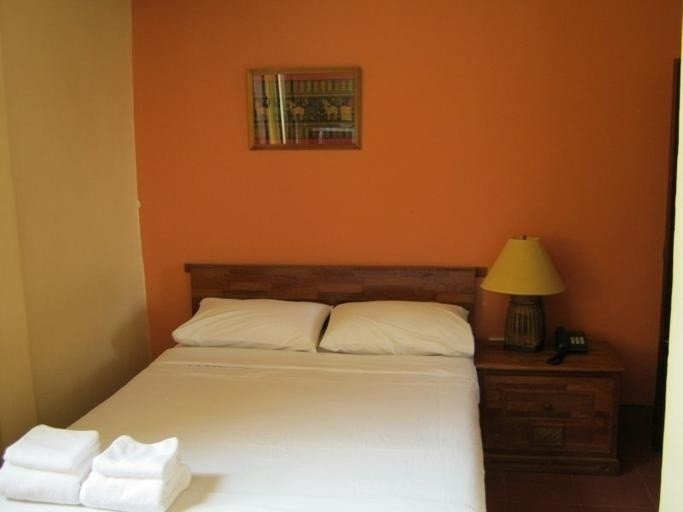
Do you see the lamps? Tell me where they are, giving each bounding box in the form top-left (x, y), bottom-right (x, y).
top-left (479, 234), bottom-right (568, 353)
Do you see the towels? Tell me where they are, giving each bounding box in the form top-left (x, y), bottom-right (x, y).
top-left (0, 423), bottom-right (193, 511)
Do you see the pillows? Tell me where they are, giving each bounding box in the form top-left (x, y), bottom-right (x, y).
top-left (170, 297), bottom-right (333, 355)
top-left (319, 300), bottom-right (476, 358)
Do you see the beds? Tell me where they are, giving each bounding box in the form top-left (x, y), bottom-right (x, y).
top-left (0, 262), bottom-right (488, 511)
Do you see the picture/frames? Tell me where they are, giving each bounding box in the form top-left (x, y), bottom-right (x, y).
top-left (246, 66), bottom-right (362, 151)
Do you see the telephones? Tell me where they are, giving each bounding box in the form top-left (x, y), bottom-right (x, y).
top-left (555, 326), bottom-right (588, 353)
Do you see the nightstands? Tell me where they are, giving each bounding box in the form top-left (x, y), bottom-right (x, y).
top-left (474, 339), bottom-right (627, 477)
